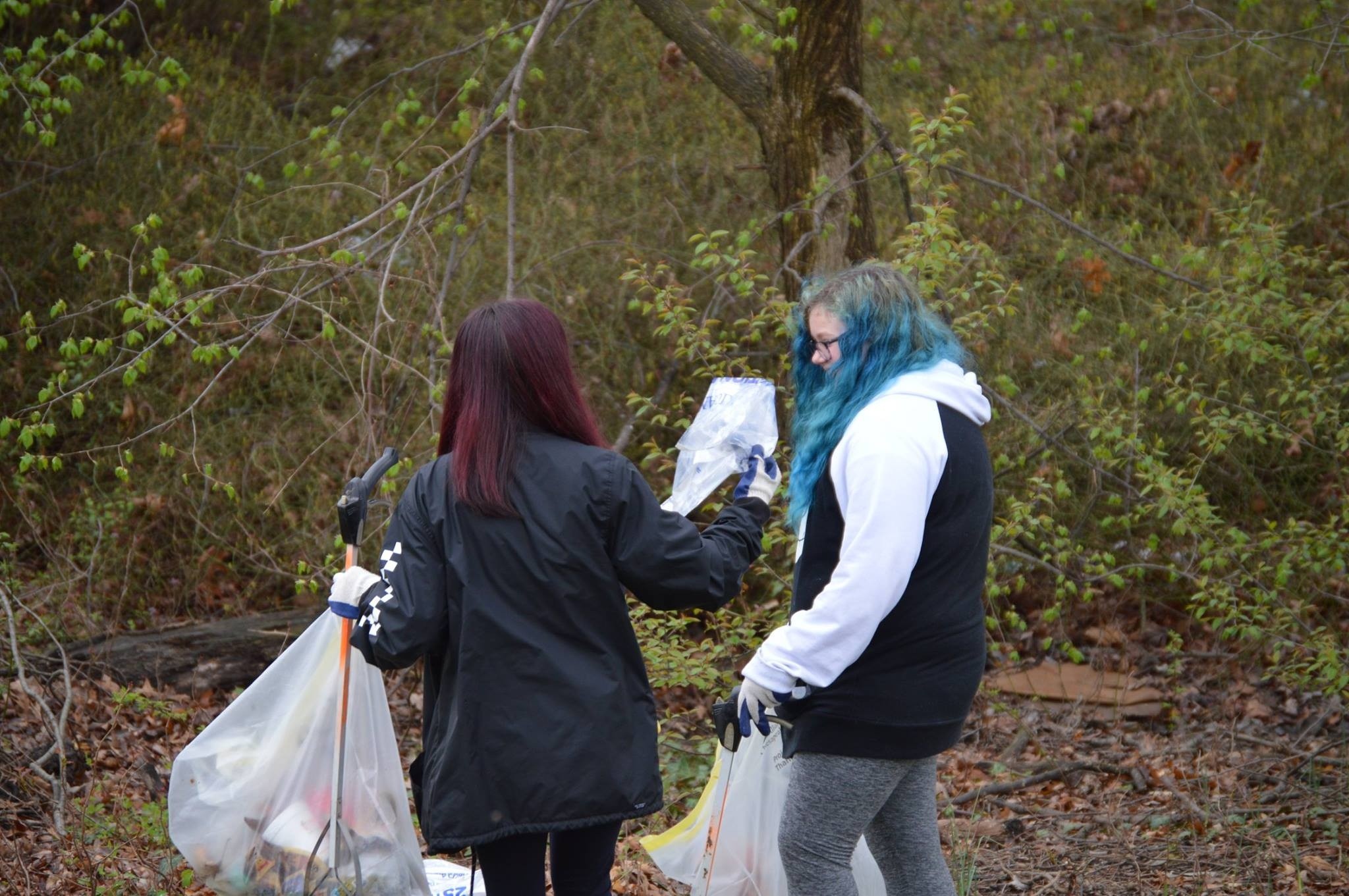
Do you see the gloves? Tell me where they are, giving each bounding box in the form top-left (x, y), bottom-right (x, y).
top-left (735, 678), bottom-right (793, 737)
top-left (732, 445), bottom-right (782, 504)
top-left (328, 566), bottom-right (382, 619)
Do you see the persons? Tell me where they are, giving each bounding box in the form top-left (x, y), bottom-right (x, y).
top-left (736, 264), bottom-right (995, 896)
top-left (327, 298), bottom-right (782, 896)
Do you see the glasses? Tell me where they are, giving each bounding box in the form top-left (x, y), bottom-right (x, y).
top-left (809, 334), bottom-right (846, 362)
